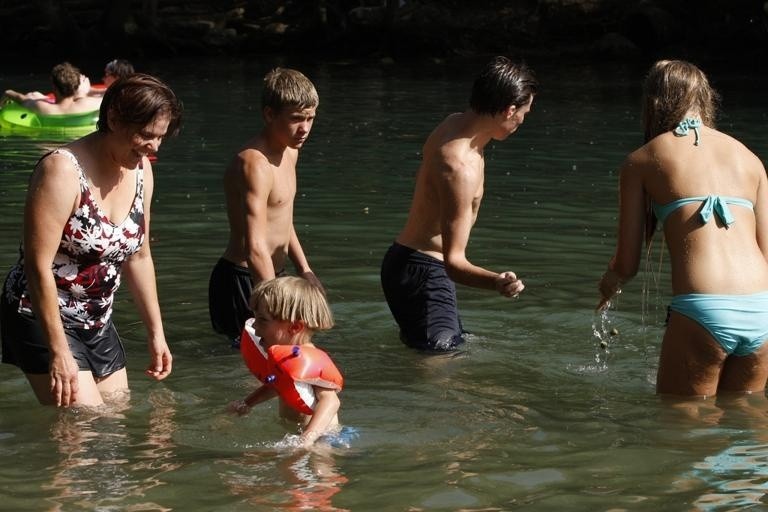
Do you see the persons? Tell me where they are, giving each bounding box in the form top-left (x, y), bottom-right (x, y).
top-left (3, 62), bottom-right (104, 116)
top-left (2, 71), bottom-right (185, 406)
top-left (594, 56), bottom-right (766, 399)
top-left (209, 67), bottom-right (328, 344)
top-left (222, 273), bottom-right (351, 462)
top-left (378, 55), bottom-right (540, 367)
top-left (88, 58), bottom-right (136, 97)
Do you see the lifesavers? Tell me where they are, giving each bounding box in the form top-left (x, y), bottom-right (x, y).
top-left (0, 83), bottom-right (111, 142)
